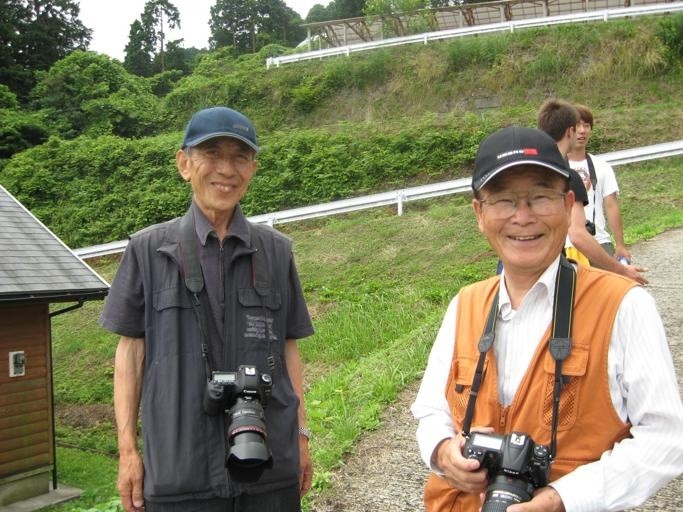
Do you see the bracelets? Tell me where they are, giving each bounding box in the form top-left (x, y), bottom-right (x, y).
top-left (297, 426), bottom-right (310, 441)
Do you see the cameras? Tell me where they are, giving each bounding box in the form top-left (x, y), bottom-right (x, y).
top-left (585, 221), bottom-right (597, 236)
top-left (205, 367), bottom-right (276, 481)
top-left (463, 430), bottom-right (553, 512)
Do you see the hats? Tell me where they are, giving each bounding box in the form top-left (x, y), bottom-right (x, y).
top-left (470, 126), bottom-right (571, 197)
top-left (181, 108), bottom-right (260, 162)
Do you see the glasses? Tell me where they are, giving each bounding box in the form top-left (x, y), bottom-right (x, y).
top-left (479, 191), bottom-right (567, 218)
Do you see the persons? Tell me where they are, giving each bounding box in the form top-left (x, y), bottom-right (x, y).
top-left (101, 105), bottom-right (316, 511)
top-left (563, 105), bottom-right (631, 272)
top-left (404, 120), bottom-right (683, 512)
top-left (535, 96), bottom-right (649, 287)
